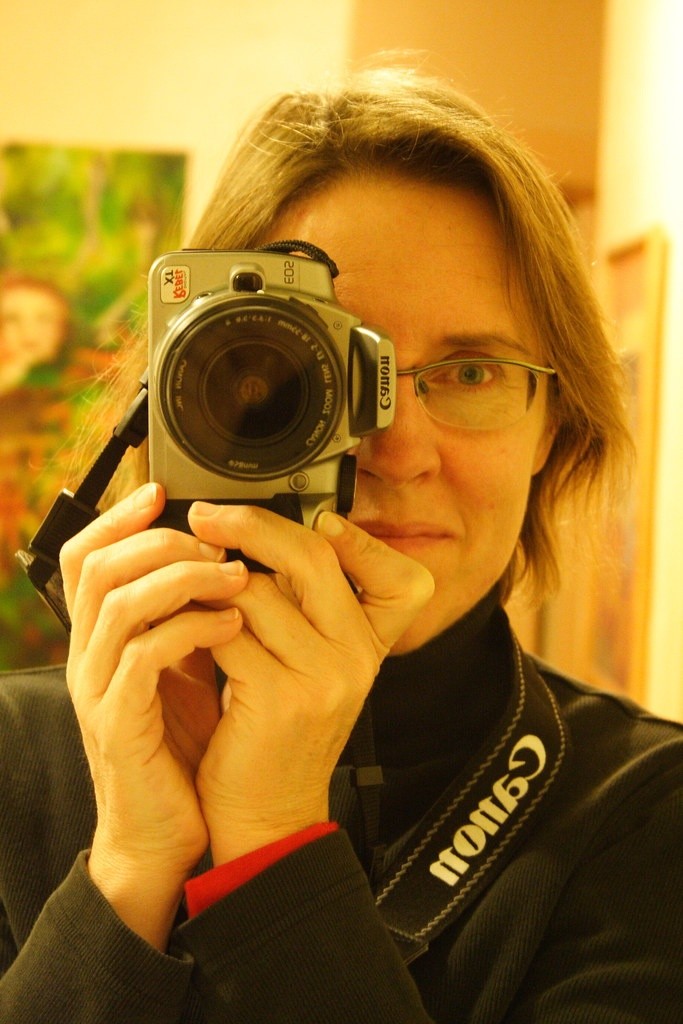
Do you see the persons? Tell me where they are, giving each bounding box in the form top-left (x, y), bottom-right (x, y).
top-left (0, 70), bottom-right (683, 1024)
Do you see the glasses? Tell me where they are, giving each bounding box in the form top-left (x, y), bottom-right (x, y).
top-left (397, 356), bottom-right (557, 432)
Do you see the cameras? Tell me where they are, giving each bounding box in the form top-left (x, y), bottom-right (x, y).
top-left (148, 243), bottom-right (400, 576)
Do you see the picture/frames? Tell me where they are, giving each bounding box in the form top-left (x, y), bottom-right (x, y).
top-left (0, 136), bottom-right (195, 668)
